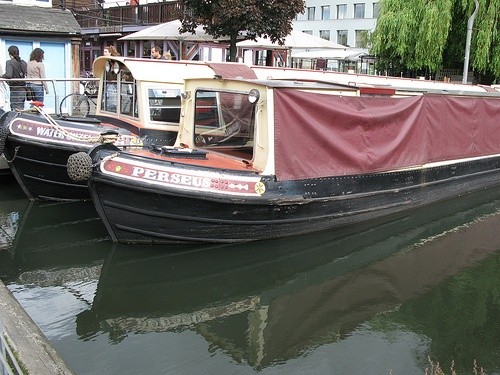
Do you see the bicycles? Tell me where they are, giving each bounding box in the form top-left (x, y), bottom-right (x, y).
top-left (61, 86), bottom-right (97, 118)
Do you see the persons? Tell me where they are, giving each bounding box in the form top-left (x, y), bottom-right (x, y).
top-left (27, 47), bottom-right (49, 110)
top-left (151, 46), bottom-right (167, 60)
top-left (0, 45), bottom-right (27, 112)
top-left (104, 46), bottom-right (120, 112)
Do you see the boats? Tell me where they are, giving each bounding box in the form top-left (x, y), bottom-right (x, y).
top-left (1, 55), bottom-right (431, 206)
top-left (83, 81), bottom-right (499, 245)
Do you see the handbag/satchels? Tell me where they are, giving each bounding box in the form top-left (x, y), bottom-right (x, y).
top-left (26, 87), bottom-right (35, 101)
